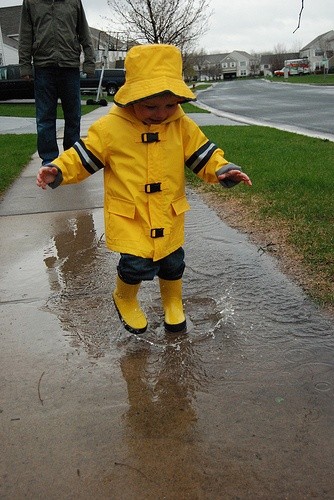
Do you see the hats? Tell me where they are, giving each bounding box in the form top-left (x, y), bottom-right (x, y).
top-left (113, 43), bottom-right (197, 107)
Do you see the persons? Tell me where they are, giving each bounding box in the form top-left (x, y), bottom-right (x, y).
top-left (18, 0), bottom-right (97, 167)
top-left (36, 44), bottom-right (253, 334)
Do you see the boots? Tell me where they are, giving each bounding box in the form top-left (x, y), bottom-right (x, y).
top-left (157, 276), bottom-right (187, 333)
top-left (113, 272), bottom-right (148, 335)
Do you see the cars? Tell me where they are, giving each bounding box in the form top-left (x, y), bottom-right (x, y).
top-left (0, 64), bottom-right (37, 101)
top-left (273, 67), bottom-right (290, 78)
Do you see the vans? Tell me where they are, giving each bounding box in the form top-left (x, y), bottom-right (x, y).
top-left (80, 68), bottom-right (126, 96)
